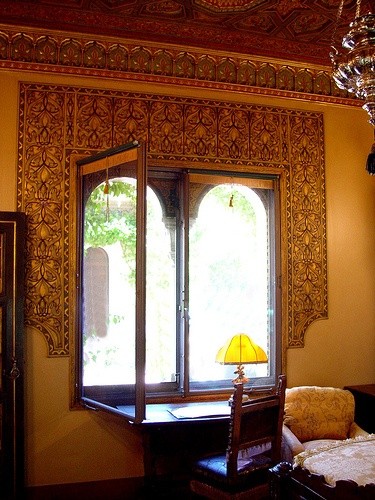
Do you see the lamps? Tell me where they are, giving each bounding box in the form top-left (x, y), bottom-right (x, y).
top-left (215, 333), bottom-right (269, 407)
top-left (329, 0), bottom-right (375, 175)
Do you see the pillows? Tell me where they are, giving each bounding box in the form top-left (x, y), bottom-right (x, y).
top-left (283, 385), bottom-right (356, 442)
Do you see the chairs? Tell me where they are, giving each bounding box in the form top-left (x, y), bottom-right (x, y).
top-left (189, 374), bottom-right (288, 500)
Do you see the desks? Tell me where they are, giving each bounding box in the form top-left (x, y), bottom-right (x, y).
top-left (116, 401), bottom-right (231, 500)
